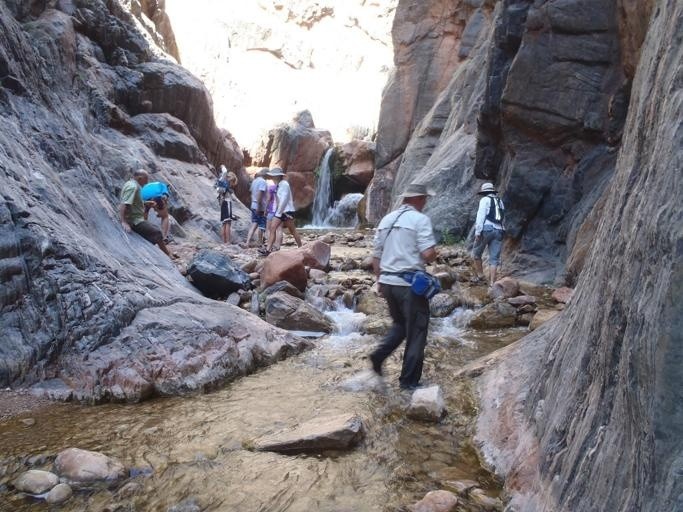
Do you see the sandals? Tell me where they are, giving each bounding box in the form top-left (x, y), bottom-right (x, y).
top-left (470, 275), bottom-right (486, 283)
top-left (257, 248), bottom-right (274, 256)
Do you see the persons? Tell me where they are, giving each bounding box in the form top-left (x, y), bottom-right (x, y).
top-left (141, 181), bottom-right (174, 245)
top-left (213, 164), bottom-right (238, 245)
top-left (471, 182), bottom-right (507, 288)
top-left (117, 168), bottom-right (169, 257)
top-left (368, 181), bottom-right (438, 390)
top-left (243, 165), bottom-right (302, 254)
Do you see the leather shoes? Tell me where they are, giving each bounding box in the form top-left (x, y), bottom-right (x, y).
top-left (400, 381), bottom-right (424, 390)
top-left (369, 351), bottom-right (385, 376)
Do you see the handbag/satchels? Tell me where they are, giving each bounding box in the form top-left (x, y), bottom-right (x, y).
top-left (400, 270), bottom-right (441, 299)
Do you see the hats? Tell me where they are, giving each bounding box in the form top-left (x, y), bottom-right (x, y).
top-left (255, 167), bottom-right (286, 176)
top-left (397, 183), bottom-right (437, 198)
top-left (475, 182), bottom-right (498, 195)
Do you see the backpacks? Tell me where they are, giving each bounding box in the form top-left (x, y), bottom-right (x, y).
top-left (484, 195), bottom-right (504, 224)
